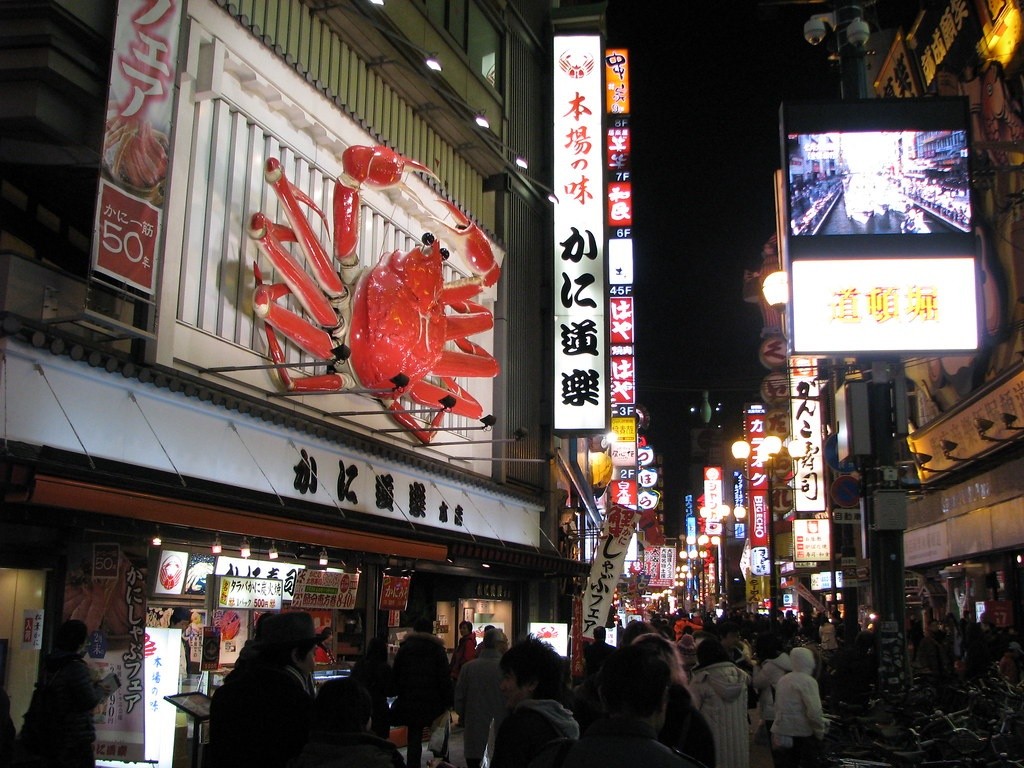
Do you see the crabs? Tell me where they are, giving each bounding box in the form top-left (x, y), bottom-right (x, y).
top-left (248, 142), bottom-right (501, 443)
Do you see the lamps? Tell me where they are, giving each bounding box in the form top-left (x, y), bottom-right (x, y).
top-left (939, 437), bottom-right (973, 462)
top-left (269, 539), bottom-right (279, 559)
top-left (425, 82), bottom-right (491, 128)
top-left (998, 413), bottom-right (1022, 429)
top-left (514, 170), bottom-right (559, 205)
top-left (203, 345), bottom-right (351, 374)
top-left (269, 372), bottom-right (411, 398)
top-left (914, 451), bottom-right (956, 474)
top-left (211, 532), bottom-right (222, 553)
top-left (153, 524), bottom-right (162, 545)
top-left (321, 393), bottom-right (456, 417)
top-left (479, 129), bottom-right (529, 170)
top-left (309, 0), bottom-right (385, 17)
top-left (319, 545), bottom-right (329, 565)
top-left (411, 427), bottom-right (530, 447)
top-left (241, 536), bottom-right (251, 557)
top-left (450, 447), bottom-right (559, 465)
top-left (974, 418), bottom-right (1010, 441)
top-left (365, 21), bottom-right (442, 73)
top-left (380, 416), bottom-right (499, 435)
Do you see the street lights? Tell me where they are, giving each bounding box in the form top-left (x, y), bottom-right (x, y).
top-left (698, 504), bottom-right (745, 611)
top-left (652, 535), bottom-right (721, 612)
top-left (730, 435), bottom-right (805, 618)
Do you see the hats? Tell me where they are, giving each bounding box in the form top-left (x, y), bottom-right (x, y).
top-left (1009, 642), bottom-right (1024, 654)
top-left (676, 634), bottom-right (696, 665)
top-left (249, 611), bottom-right (332, 651)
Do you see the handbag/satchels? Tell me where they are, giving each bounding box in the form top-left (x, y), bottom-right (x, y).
top-left (19, 690), bottom-right (49, 755)
top-left (770, 732), bottom-right (793, 751)
top-left (753, 719), bottom-right (770, 746)
top-left (427, 711), bottom-right (450, 759)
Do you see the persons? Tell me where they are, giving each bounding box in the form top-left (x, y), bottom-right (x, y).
top-left (313, 625), bottom-right (337, 663)
top-left (0, 605), bottom-right (1018, 768)
top-left (771, 646), bottom-right (835, 768)
top-left (210, 612), bottom-right (319, 768)
top-left (18, 620), bottom-right (111, 768)
top-left (489, 633), bottom-right (574, 768)
top-left (688, 640), bottom-right (753, 768)
top-left (393, 618), bottom-right (454, 768)
top-left (534, 644), bottom-right (708, 768)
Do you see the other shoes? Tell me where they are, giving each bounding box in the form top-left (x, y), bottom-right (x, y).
top-left (454, 719), bottom-right (464, 727)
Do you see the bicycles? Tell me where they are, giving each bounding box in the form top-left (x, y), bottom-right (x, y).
top-left (821, 661), bottom-right (1024, 768)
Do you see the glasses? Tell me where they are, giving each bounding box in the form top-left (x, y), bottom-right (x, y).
top-left (727, 632), bottom-right (742, 641)
top-left (492, 637), bottom-right (509, 646)
